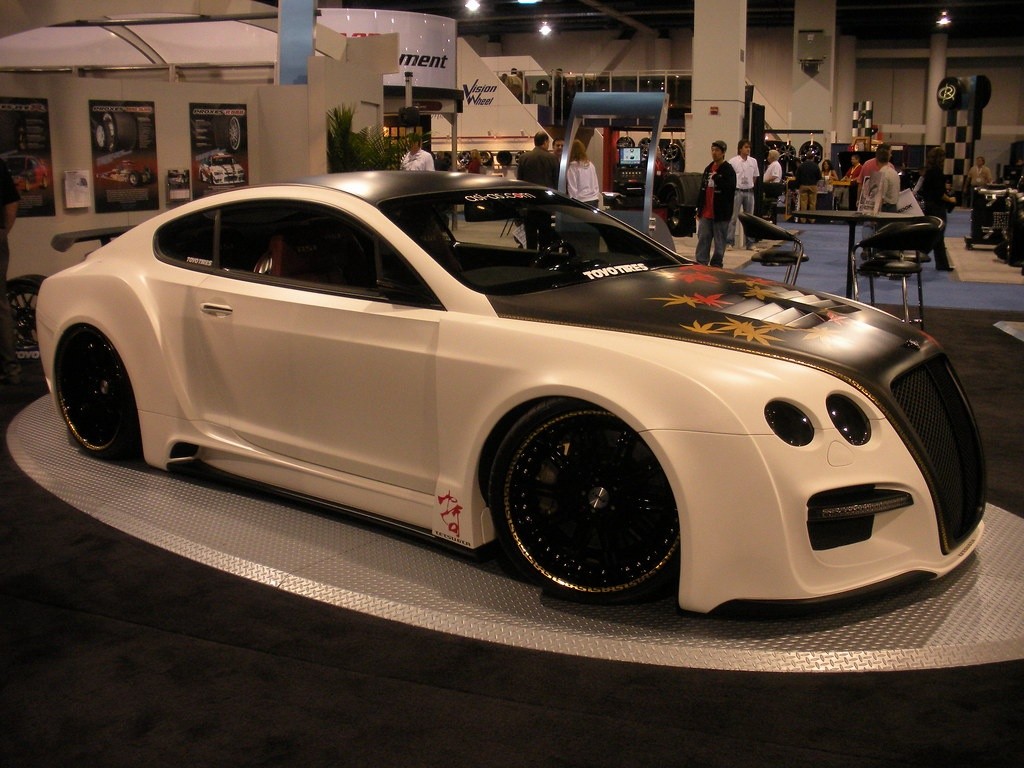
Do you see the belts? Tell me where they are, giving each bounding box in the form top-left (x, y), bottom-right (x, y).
top-left (736, 188), bottom-right (753, 192)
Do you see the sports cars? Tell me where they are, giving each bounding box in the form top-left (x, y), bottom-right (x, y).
top-left (199, 154), bottom-right (247, 186)
top-left (95, 159), bottom-right (155, 187)
top-left (1, 154), bottom-right (50, 193)
top-left (32, 168), bottom-right (988, 619)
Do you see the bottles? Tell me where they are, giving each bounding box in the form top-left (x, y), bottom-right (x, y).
top-left (828, 176), bottom-right (834, 192)
top-left (840, 176), bottom-right (849, 184)
top-left (945, 180), bottom-right (951, 193)
top-left (947, 196), bottom-right (958, 213)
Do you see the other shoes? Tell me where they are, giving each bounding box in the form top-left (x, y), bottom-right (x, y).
top-left (938, 266), bottom-right (954, 271)
top-left (726, 244), bottom-right (734, 251)
top-left (748, 245), bottom-right (756, 250)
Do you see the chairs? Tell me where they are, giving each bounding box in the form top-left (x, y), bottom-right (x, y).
top-left (737, 212), bottom-right (809, 286)
top-left (862, 215), bottom-right (945, 316)
top-left (849, 224), bottom-right (936, 330)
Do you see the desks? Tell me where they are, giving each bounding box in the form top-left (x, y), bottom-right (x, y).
top-left (791, 209), bottom-right (913, 252)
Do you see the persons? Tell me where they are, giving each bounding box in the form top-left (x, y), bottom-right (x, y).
top-left (517, 131), bottom-right (560, 249)
top-left (796, 151), bottom-right (821, 224)
top-left (400, 133), bottom-right (434, 171)
top-left (550, 136), bottom-right (564, 159)
top-left (642, 145), bottom-right (672, 193)
top-left (428, 151), bottom-right (450, 171)
top-left (693, 140), bottom-right (737, 268)
top-left (964, 156), bottom-right (992, 209)
top-left (763, 150), bottom-right (782, 223)
top-left (875, 149), bottom-right (900, 231)
top-left (0, 156), bottom-right (22, 385)
top-left (913, 147), bottom-right (957, 271)
top-left (857, 143), bottom-right (895, 259)
top-left (842, 154), bottom-right (863, 211)
top-left (465, 149), bottom-right (482, 174)
top-left (566, 139), bottom-right (600, 208)
top-left (821, 160), bottom-right (838, 185)
top-left (725, 140), bottom-right (760, 251)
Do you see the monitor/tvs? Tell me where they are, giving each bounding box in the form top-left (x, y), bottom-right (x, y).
top-left (619, 147), bottom-right (642, 168)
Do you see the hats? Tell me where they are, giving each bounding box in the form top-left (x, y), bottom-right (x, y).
top-left (713, 140), bottom-right (727, 159)
